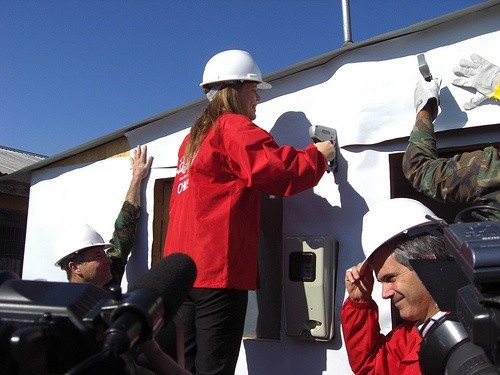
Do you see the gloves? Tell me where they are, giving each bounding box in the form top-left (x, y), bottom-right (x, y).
top-left (414, 76), bottom-right (443, 121)
top-left (451, 52), bottom-right (500, 110)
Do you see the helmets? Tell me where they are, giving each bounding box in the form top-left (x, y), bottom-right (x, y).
top-left (52, 221), bottom-right (115, 268)
top-left (200, 49), bottom-right (273, 90)
top-left (359, 198), bottom-right (445, 276)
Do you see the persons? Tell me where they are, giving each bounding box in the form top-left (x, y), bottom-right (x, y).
top-left (153, 50), bottom-right (337, 374)
top-left (53, 145), bottom-right (154, 292)
top-left (340, 198), bottom-right (475, 374)
top-left (402, 52), bottom-right (500, 223)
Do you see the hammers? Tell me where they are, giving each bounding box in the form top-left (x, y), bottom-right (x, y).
top-left (417, 53), bottom-right (441, 106)
